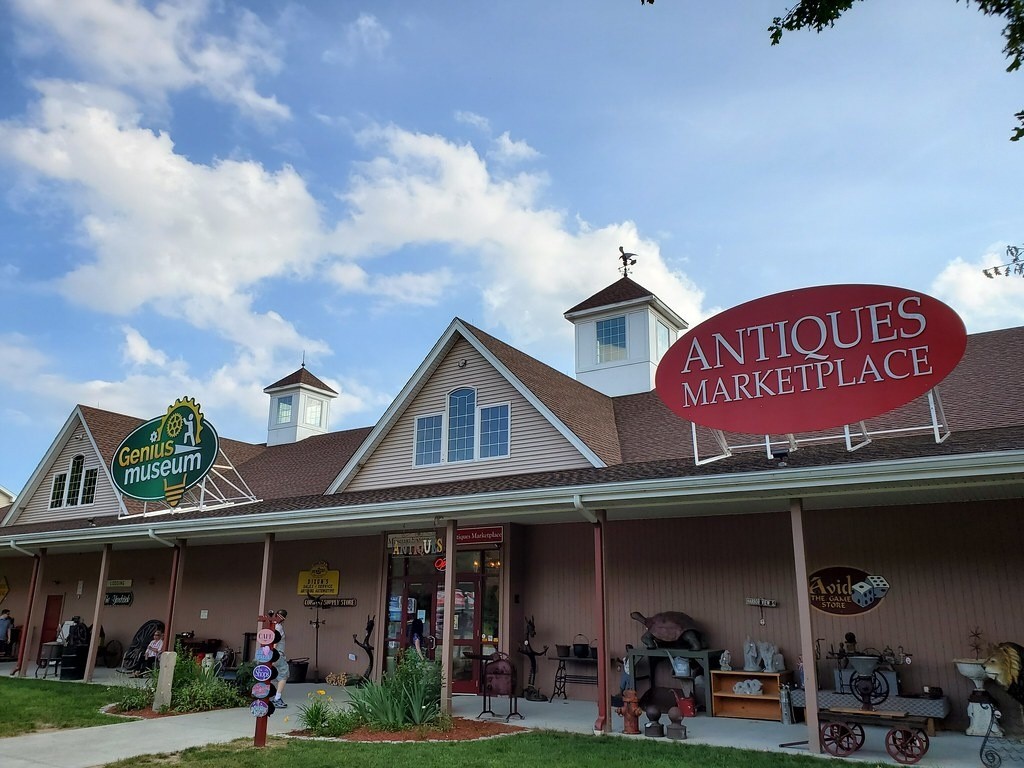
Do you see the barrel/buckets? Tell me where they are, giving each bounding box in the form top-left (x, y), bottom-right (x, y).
top-left (590, 638), bottom-right (598, 659)
top-left (555, 643), bottom-right (572, 658)
top-left (285, 656), bottom-right (310, 683)
top-left (60, 644), bottom-right (88, 681)
top-left (572, 634), bottom-right (591, 658)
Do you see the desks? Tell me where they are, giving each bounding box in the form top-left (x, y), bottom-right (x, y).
top-left (546, 656), bottom-right (598, 703)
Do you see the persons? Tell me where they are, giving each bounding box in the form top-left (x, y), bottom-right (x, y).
top-left (0, 609), bottom-right (11, 652)
top-left (132, 631), bottom-right (164, 678)
top-left (273, 610), bottom-right (290, 708)
top-left (408, 618), bottom-right (427, 662)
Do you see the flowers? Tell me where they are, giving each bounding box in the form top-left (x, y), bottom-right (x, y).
top-left (283, 690), bottom-right (333, 729)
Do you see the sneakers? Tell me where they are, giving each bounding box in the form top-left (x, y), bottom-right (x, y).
top-left (272, 698), bottom-right (288, 708)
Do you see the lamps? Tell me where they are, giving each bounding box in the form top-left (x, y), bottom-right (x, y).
top-left (473, 554), bottom-right (502, 571)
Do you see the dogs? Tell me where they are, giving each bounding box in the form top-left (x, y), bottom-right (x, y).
top-left (980, 642), bottom-right (1024, 706)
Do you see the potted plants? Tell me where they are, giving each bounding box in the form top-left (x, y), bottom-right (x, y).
top-left (954, 624), bottom-right (1009, 740)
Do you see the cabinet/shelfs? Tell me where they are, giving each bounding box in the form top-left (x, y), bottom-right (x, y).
top-left (710, 669), bottom-right (795, 723)
